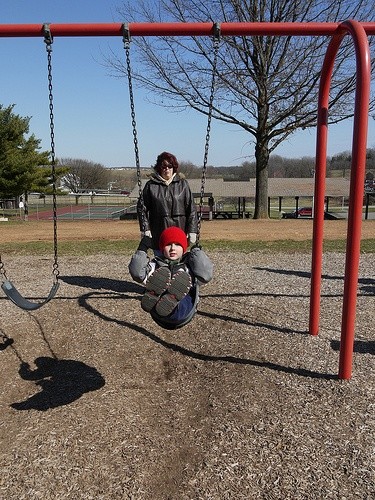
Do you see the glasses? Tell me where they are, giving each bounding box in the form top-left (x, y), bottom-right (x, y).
top-left (160, 164), bottom-right (173, 170)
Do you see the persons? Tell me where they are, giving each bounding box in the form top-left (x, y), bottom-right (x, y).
top-left (208, 197), bottom-right (214, 211)
top-left (128, 227), bottom-right (212, 330)
top-left (24, 201), bottom-right (28, 222)
top-left (137, 152), bottom-right (199, 258)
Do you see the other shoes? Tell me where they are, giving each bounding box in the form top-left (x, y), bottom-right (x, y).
top-left (156, 271), bottom-right (192, 317)
top-left (141, 265), bottom-right (172, 311)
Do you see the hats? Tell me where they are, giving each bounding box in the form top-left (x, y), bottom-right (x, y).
top-left (159, 227), bottom-right (187, 255)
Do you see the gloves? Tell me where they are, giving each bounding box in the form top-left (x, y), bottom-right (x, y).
top-left (138, 236), bottom-right (151, 253)
top-left (186, 232), bottom-right (196, 244)
top-left (141, 230), bottom-right (152, 238)
top-left (190, 241), bottom-right (202, 250)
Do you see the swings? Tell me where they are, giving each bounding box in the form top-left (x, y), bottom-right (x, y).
top-left (0, 22), bottom-right (60, 312)
top-left (121, 18), bottom-right (222, 330)
